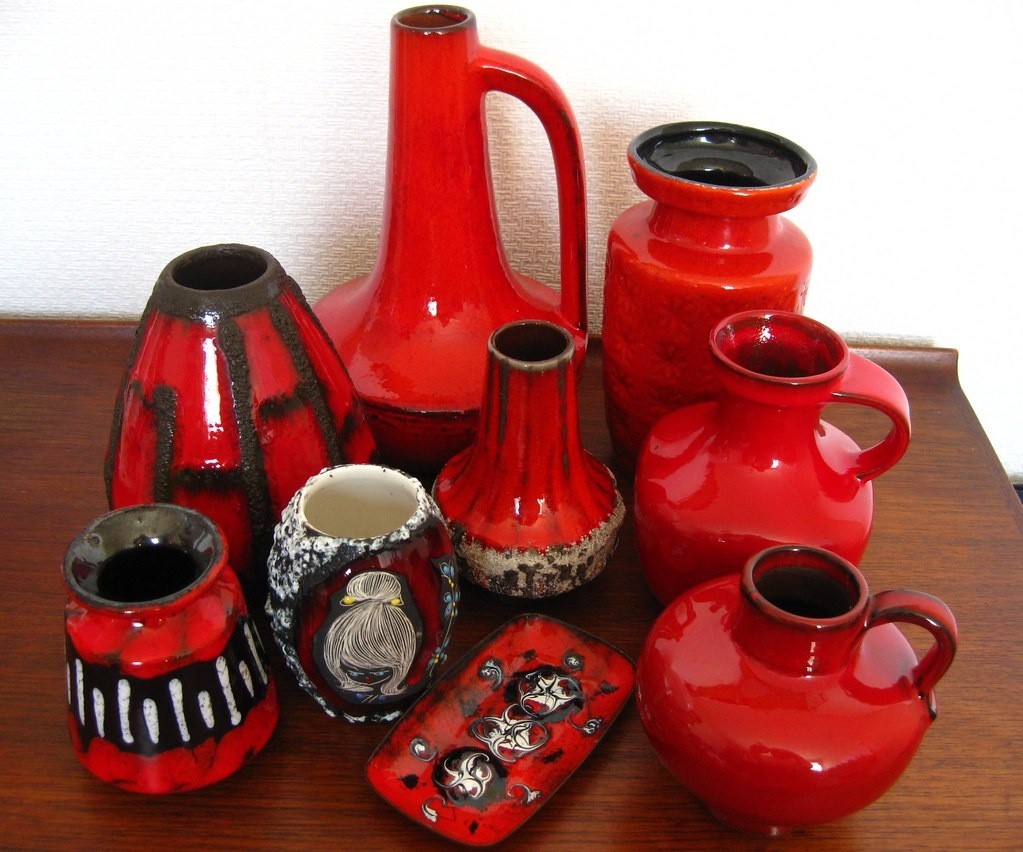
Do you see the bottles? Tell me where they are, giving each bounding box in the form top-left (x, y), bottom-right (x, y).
top-left (431, 318), bottom-right (626, 598)
top-left (100, 243), bottom-right (377, 603)
top-left (61, 501), bottom-right (281, 797)
top-left (310, 4), bottom-right (589, 483)
top-left (267, 458), bottom-right (461, 725)
top-left (602, 119), bottom-right (819, 465)
top-left (634, 543), bottom-right (959, 838)
top-left (633, 309), bottom-right (913, 602)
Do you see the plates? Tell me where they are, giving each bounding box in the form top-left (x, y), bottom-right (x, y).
top-left (363, 611), bottom-right (638, 850)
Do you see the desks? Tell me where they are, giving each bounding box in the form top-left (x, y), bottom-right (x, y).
top-left (0, 318), bottom-right (1023, 852)
